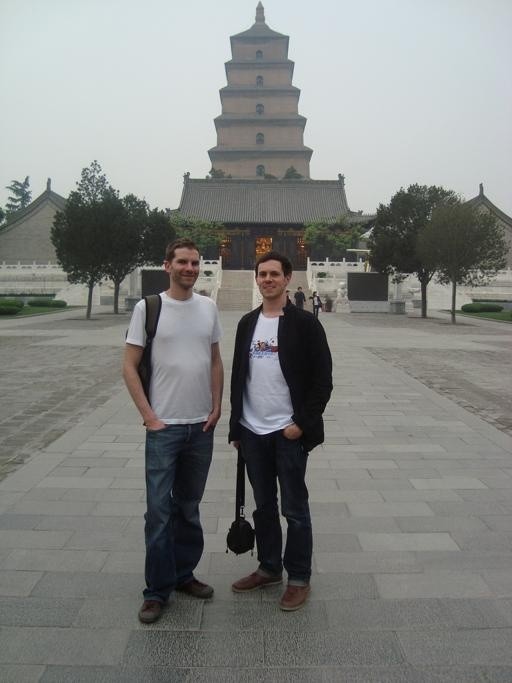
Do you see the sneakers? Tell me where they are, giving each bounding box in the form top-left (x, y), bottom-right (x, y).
top-left (176, 579), bottom-right (213, 599)
top-left (280, 585), bottom-right (310, 611)
top-left (232, 572), bottom-right (282, 592)
top-left (139, 600), bottom-right (163, 623)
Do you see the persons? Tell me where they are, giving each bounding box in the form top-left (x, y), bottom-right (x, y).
top-left (119, 237), bottom-right (224, 628)
top-left (308, 290), bottom-right (323, 317)
top-left (293, 286), bottom-right (306, 309)
top-left (229, 252), bottom-right (334, 612)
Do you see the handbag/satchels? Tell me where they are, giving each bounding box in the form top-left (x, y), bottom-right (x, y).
top-left (227, 518), bottom-right (255, 553)
top-left (126, 295), bottom-right (162, 405)
